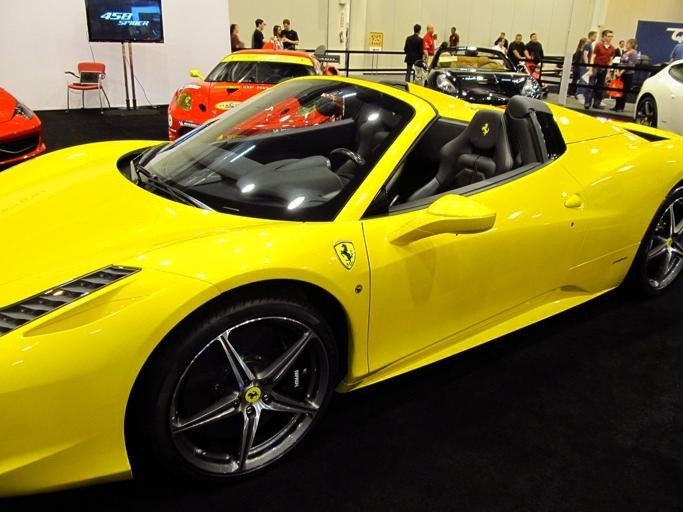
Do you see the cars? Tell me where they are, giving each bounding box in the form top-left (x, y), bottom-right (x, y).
top-left (632, 59), bottom-right (683, 136)
top-left (0, 86), bottom-right (48, 171)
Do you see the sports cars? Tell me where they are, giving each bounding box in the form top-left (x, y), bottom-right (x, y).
top-left (166, 49), bottom-right (340, 145)
top-left (408, 46), bottom-right (545, 108)
top-left (0, 74), bottom-right (683, 498)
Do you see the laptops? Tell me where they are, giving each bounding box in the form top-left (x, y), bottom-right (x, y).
top-left (73, 71), bottom-right (101, 85)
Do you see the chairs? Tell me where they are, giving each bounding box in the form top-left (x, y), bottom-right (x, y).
top-left (335, 80), bottom-right (410, 189)
top-left (64, 63), bottom-right (110, 115)
top-left (405, 109), bottom-right (515, 202)
top-left (437, 51), bottom-right (508, 70)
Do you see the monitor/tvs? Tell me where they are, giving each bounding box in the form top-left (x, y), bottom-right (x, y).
top-left (85, 0), bottom-right (164, 43)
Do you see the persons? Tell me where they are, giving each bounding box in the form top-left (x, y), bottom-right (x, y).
top-left (279, 18), bottom-right (299, 51)
top-left (251, 18), bottom-right (265, 49)
top-left (271, 25), bottom-right (285, 50)
top-left (230, 23), bottom-right (245, 53)
top-left (403, 23), bottom-right (683, 113)
